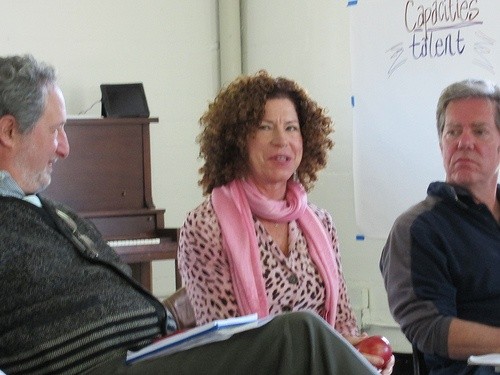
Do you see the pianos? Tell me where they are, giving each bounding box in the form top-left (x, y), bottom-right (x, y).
top-left (41, 117), bottom-right (183, 304)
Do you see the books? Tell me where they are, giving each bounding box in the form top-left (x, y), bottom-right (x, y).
top-left (124, 313), bottom-right (260, 365)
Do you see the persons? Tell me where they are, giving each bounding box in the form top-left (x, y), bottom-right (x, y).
top-left (380, 79), bottom-right (500, 375)
top-left (176, 70), bottom-right (395, 375)
top-left (0, 54), bottom-right (383, 375)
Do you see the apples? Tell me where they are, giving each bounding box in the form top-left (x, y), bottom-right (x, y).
top-left (354, 335), bottom-right (392, 368)
